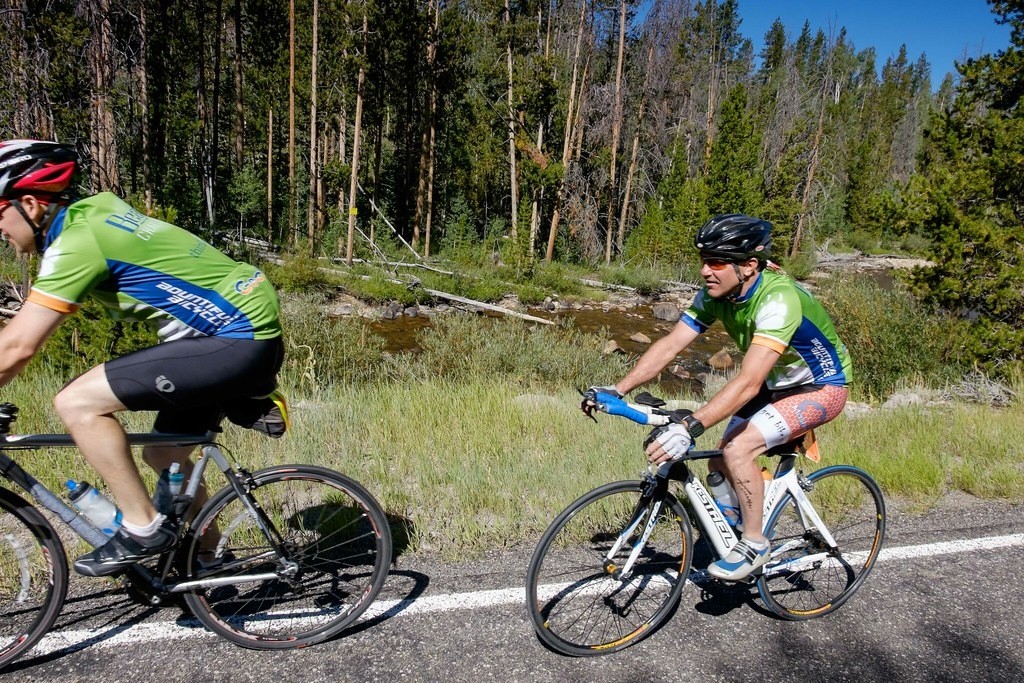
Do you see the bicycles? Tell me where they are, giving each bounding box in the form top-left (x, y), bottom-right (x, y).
top-left (0, 378), bottom-right (394, 671)
top-left (525, 387), bottom-right (887, 657)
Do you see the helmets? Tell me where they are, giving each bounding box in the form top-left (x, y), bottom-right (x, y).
top-left (694, 213), bottom-right (772, 255)
top-left (0, 138), bottom-right (79, 199)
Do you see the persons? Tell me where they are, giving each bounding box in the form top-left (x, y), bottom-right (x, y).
top-left (577, 214), bottom-right (853, 581)
top-left (0, 139), bottom-right (285, 578)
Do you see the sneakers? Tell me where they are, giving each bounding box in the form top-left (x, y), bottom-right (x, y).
top-left (707, 539), bottom-right (771, 581)
top-left (196, 551), bottom-right (234, 580)
top-left (74, 515), bottom-right (178, 577)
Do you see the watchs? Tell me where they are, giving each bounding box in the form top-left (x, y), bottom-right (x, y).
top-left (682, 415), bottom-right (705, 438)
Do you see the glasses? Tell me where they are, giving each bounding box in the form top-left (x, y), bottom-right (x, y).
top-left (700, 254), bottom-right (742, 271)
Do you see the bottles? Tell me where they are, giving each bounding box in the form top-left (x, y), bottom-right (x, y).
top-left (761, 467), bottom-right (773, 498)
top-left (706, 469), bottom-right (742, 525)
top-left (152, 463), bottom-right (185, 513)
top-left (65, 480), bottom-right (123, 538)
